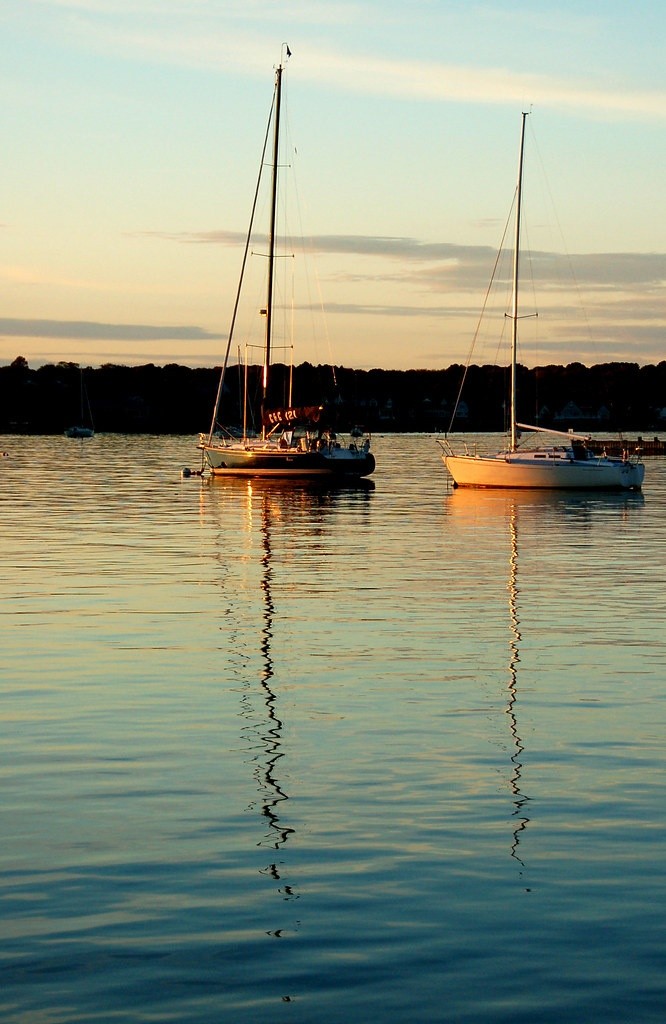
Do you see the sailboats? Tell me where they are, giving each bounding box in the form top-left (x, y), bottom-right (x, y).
top-left (436, 104), bottom-right (645, 491)
top-left (63, 365), bottom-right (96, 438)
top-left (195, 41), bottom-right (375, 478)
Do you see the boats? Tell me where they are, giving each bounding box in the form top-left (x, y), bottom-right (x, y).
top-left (569, 435), bottom-right (666, 456)
